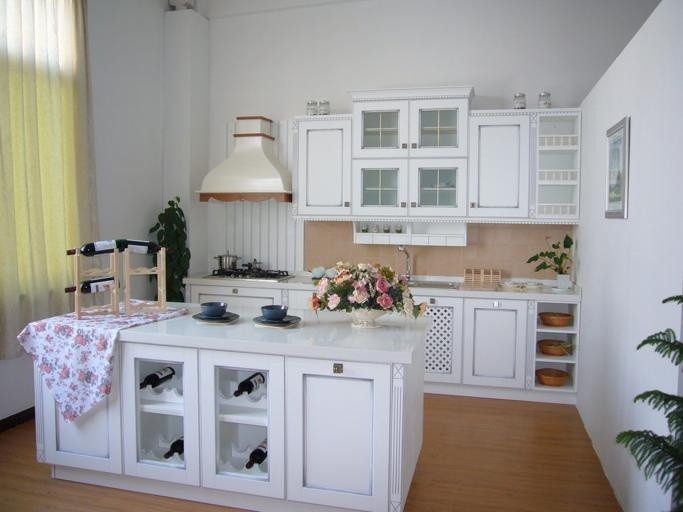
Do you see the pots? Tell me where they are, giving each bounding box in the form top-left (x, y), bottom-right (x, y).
top-left (213, 249), bottom-right (241, 269)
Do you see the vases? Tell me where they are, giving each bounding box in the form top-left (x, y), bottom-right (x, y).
top-left (344, 307), bottom-right (387, 329)
top-left (557, 274), bottom-right (570, 289)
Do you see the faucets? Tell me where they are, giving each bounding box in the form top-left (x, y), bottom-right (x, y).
top-left (397, 245), bottom-right (412, 281)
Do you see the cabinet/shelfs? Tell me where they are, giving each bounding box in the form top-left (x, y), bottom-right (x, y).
top-left (532, 301), bottom-right (581, 393)
top-left (469, 110), bottom-right (536, 224)
top-left (353, 221), bottom-right (466, 247)
top-left (536, 107), bottom-right (582, 224)
top-left (292, 114), bottom-right (351, 221)
top-left (29, 298), bottom-right (433, 512)
top-left (189, 285), bottom-right (282, 310)
top-left (287, 290), bottom-right (463, 389)
top-left (462, 298), bottom-right (528, 388)
top-left (346, 86), bottom-right (475, 223)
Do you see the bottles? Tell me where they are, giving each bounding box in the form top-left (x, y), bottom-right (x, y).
top-left (512, 92), bottom-right (526, 109)
top-left (164, 435), bottom-right (184, 459)
top-left (245, 436), bottom-right (267, 469)
top-left (537, 92), bottom-right (551, 108)
top-left (140, 366), bottom-right (176, 389)
top-left (64, 275), bottom-right (121, 294)
top-left (66, 239), bottom-right (124, 257)
top-left (124, 239), bottom-right (175, 255)
top-left (233, 372), bottom-right (265, 397)
top-left (306, 100), bottom-right (318, 115)
top-left (319, 100), bottom-right (330, 115)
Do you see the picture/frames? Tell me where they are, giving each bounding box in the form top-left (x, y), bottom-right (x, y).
top-left (604, 116), bottom-right (628, 219)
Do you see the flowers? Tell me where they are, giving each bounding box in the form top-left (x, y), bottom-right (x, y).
top-left (527, 233), bottom-right (574, 274)
top-left (310, 260), bottom-right (428, 320)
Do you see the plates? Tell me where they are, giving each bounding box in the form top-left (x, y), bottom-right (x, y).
top-left (192, 311), bottom-right (240, 326)
top-left (252, 315), bottom-right (301, 328)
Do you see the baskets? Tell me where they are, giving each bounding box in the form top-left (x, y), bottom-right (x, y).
top-left (537, 339), bottom-right (566, 355)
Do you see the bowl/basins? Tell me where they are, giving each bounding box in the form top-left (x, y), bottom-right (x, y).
top-left (261, 305), bottom-right (288, 322)
top-left (538, 340), bottom-right (571, 356)
top-left (200, 301), bottom-right (227, 316)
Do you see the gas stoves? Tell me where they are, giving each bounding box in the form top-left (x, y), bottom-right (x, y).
top-left (211, 267), bottom-right (288, 279)
